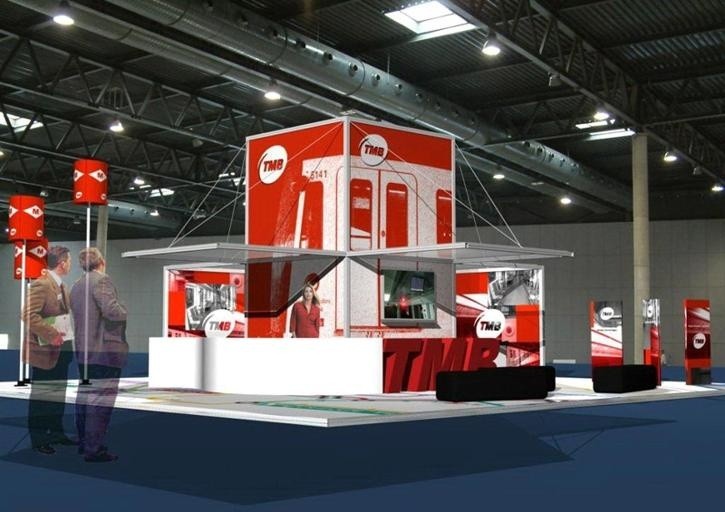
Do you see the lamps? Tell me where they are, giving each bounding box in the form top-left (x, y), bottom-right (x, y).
top-left (0, 0), bottom-right (725, 217)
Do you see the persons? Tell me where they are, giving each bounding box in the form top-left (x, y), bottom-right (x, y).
top-left (291, 283), bottom-right (323, 337)
top-left (67, 247), bottom-right (130, 466)
top-left (18, 246), bottom-right (80, 455)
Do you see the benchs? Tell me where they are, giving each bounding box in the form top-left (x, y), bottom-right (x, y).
top-left (591, 365), bottom-right (659, 394)
top-left (435, 365), bottom-right (556, 404)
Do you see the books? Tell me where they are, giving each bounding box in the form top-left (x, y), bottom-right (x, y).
top-left (36, 312), bottom-right (74, 347)
